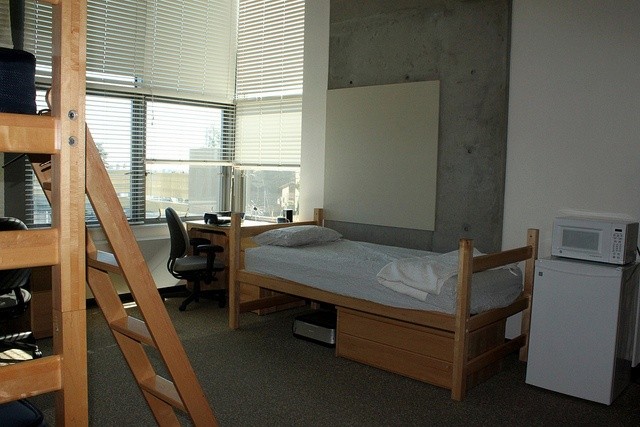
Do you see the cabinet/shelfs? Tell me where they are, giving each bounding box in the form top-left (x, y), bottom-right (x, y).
top-left (26, 280), bottom-right (52, 340)
top-left (334, 302), bottom-right (508, 391)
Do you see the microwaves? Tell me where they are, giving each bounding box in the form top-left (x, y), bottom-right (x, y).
top-left (551, 215), bottom-right (638, 264)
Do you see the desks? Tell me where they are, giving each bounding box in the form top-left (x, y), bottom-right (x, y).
top-left (184, 218), bottom-right (308, 315)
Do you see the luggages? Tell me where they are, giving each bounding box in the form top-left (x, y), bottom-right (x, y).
top-left (292, 311), bottom-right (337, 348)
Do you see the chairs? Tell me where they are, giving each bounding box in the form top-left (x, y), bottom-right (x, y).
top-left (0, 214), bottom-right (42, 359)
top-left (165, 206), bottom-right (227, 311)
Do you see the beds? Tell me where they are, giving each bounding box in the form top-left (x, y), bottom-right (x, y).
top-left (1, 0), bottom-right (87, 426)
top-left (228, 207), bottom-right (539, 401)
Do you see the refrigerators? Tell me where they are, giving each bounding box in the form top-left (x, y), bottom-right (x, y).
top-left (524, 256), bottom-right (639, 405)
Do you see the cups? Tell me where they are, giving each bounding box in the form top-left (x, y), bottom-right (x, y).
top-left (277, 216), bottom-right (290, 223)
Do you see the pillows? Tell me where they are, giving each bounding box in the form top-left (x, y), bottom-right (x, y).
top-left (250, 224), bottom-right (343, 246)
